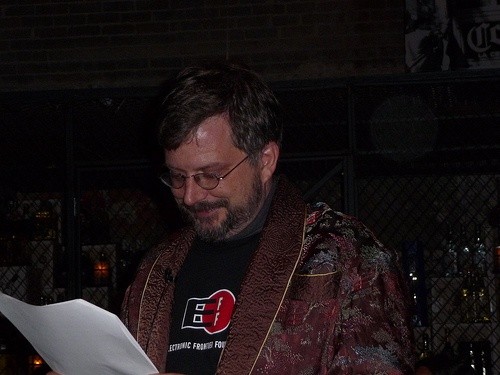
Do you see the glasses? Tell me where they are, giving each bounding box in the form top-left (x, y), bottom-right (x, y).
top-left (159, 153), bottom-right (253, 191)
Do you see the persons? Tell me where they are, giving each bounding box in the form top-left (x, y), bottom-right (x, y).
top-left (45, 61), bottom-right (430, 375)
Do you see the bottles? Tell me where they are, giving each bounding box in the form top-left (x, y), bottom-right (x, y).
top-left (443, 221), bottom-right (488, 278)
top-left (418, 326), bottom-right (492, 375)
top-left (457, 276), bottom-right (490, 323)
